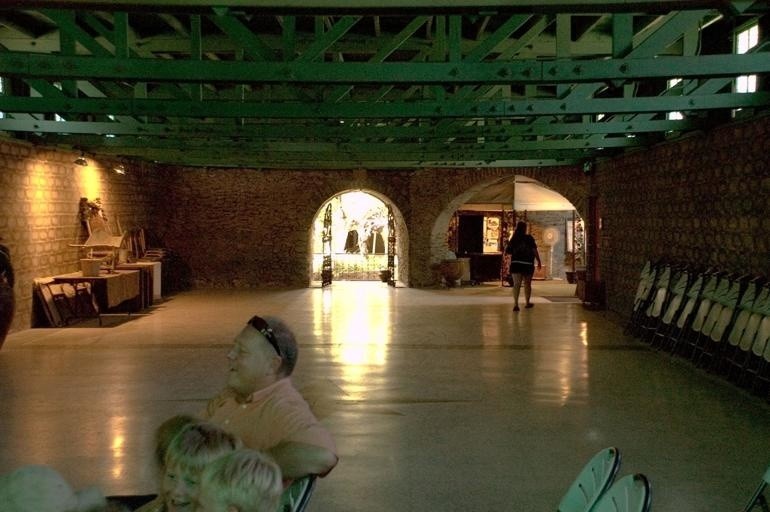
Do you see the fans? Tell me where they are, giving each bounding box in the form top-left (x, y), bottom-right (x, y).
top-left (542, 225), bottom-right (560, 280)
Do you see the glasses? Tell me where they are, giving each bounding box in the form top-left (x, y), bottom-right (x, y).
top-left (248, 314), bottom-right (280, 357)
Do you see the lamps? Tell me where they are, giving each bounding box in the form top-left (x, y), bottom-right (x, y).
top-left (74, 151), bottom-right (90, 167)
top-left (112, 155), bottom-right (129, 176)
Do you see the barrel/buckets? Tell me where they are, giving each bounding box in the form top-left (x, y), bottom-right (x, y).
top-left (80, 259), bottom-right (102, 277)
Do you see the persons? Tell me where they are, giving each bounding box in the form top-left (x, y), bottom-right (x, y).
top-left (197, 317), bottom-right (338, 481)
top-left (0, 464), bottom-right (117, 512)
top-left (195, 448), bottom-right (285, 512)
top-left (162, 422), bottom-right (243, 510)
top-left (504, 221), bottom-right (541, 313)
top-left (133, 413), bottom-right (200, 510)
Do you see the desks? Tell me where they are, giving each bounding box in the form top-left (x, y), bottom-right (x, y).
top-left (115, 262), bottom-right (163, 310)
top-left (52, 269), bottom-right (141, 326)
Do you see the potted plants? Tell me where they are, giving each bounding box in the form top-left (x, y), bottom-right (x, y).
top-left (562, 250), bottom-right (576, 284)
top-left (573, 212), bottom-right (587, 299)
top-left (80, 248), bottom-right (102, 277)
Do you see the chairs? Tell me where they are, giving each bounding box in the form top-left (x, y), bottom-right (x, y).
top-left (744, 466), bottom-right (770, 512)
top-left (276, 474), bottom-right (320, 512)
top-left (558, 445), bottom-right (623, 512)
top-left (623, 256), bottom-right (770, 404)
top-left (589, 470), bottom-right (652, 512)
